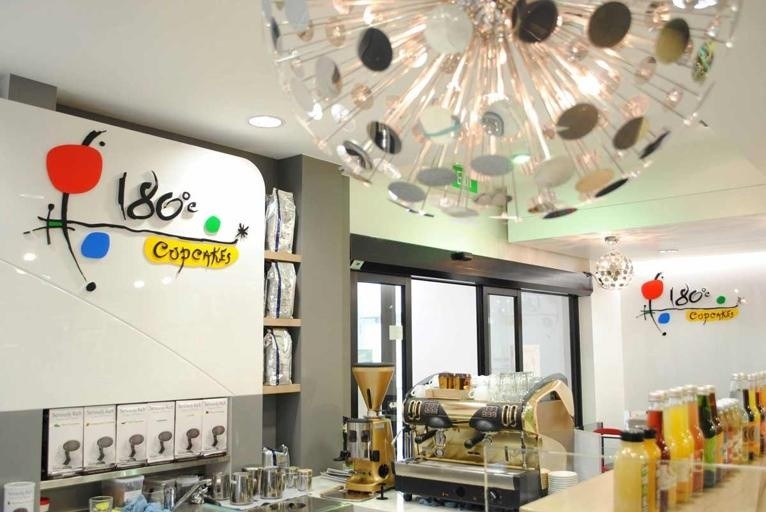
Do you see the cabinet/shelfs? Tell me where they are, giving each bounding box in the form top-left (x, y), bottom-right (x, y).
top-left (260, 249), bottom-right (302, 397)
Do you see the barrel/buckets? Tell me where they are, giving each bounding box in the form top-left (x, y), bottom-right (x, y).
top-left (4, 481), bottom-right (35, 512)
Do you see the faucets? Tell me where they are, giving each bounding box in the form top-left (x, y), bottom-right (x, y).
top-left (164, 478), bottom-right (213, 512)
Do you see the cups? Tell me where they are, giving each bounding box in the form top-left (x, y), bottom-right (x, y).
top-left (90, 495), bottom-right (114, 511)
top-left (409, 371), bottom-right (544, 404)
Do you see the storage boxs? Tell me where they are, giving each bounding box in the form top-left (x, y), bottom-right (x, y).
top-left (36, 398), bottom-right (228, 476)
top-left (100, 476), bottom-right (142, 508)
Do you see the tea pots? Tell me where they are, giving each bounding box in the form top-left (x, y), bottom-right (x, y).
top-left (209, 464), bottom-right (312, 506)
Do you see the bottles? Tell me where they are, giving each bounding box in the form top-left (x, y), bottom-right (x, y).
top-left (40, 496), bottom-right (50, 512)
top-left (612, 369), bottom-right (766, 512)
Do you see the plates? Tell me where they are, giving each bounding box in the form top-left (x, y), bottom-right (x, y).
top-left (546, 470), bottom-right (579, 497)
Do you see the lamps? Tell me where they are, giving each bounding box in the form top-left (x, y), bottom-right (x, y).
top-left (253, 0), bottom-right (746, 237)
top-left (591, 235), bottom-right (634, 291)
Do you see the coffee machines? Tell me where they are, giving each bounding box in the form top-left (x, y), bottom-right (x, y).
top-left (395, 373), bottom-right (576, 511)
top-left (344, 361), bottom-right (397, 495)
